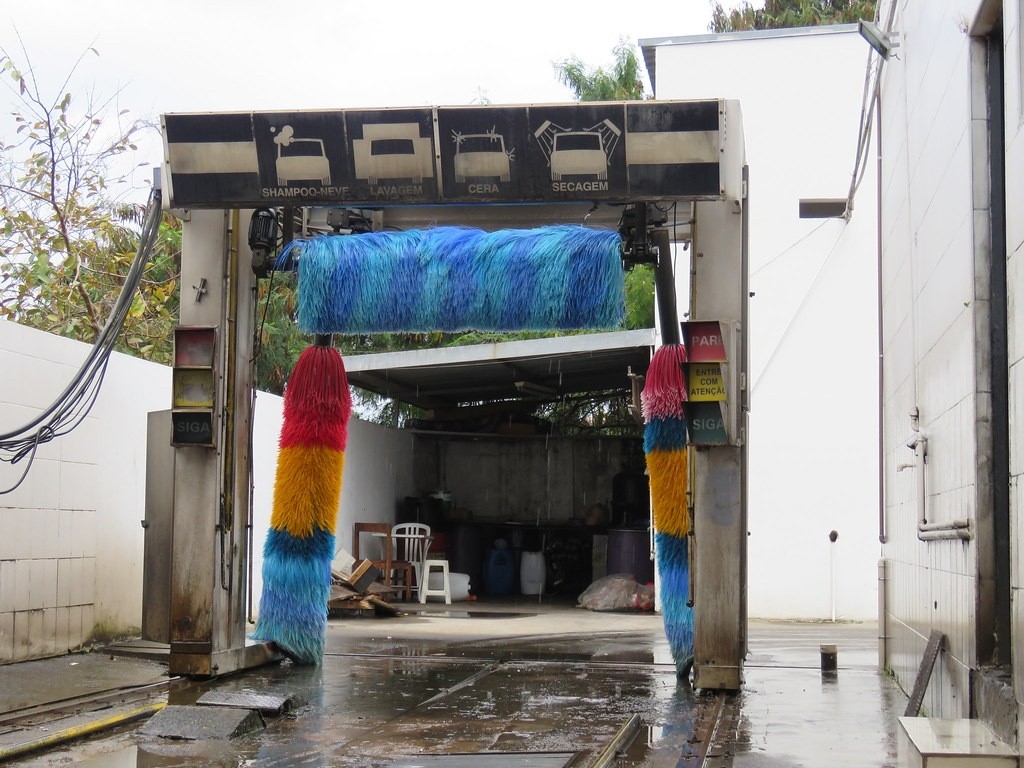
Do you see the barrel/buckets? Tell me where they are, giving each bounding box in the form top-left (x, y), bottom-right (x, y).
top-left (401, 494), bottom-right (514, 601)
top-left (607, 528), bottom-right (653, 585)
top-left (519, 550), bottom-right (547, 594)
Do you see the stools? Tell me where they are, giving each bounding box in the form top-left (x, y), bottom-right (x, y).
top-left (420, 560), bottom-right (451, 604)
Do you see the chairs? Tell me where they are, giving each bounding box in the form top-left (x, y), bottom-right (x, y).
top-left (392, 523), bottom-right (431, 601)
top-left (354, 523), bottom-right (412, 601)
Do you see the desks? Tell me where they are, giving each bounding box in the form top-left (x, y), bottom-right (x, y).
top-left (372, 534), bottom-right (434, 601)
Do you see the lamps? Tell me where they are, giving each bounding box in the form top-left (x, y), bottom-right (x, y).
top-left (858, 18), bottom-right (900, 61)
top-left (799, 198), bottom-right (854, 219)
top-left (515, 381), bottom-right (557, 396)
